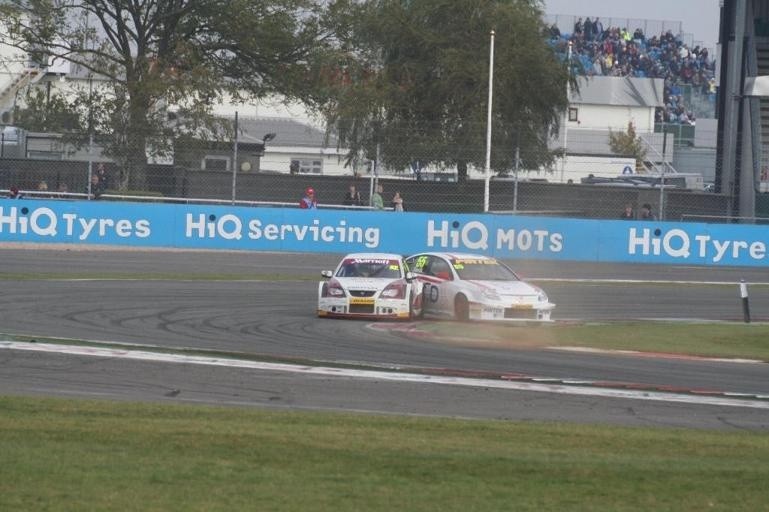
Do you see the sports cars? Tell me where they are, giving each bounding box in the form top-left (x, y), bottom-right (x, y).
top-left (406, 251), bottom-right (558, 324)
top-left (315, 252), bottom-right (425, 321)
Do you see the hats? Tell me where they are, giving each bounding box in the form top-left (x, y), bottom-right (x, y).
top-left (306, 187), bottom-right (316, 194)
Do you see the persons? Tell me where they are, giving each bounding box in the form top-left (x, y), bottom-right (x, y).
top-left (392, 192), bottom-right (406, 211)
top-left (372, 185), bottom-right (386, 208)
top-left (639, 203), bottom-right (658, 221)
top-left (620, 203), bottom-right (634, 220)
top-left (543, 17), bottom-right (717, 124)
top-left (343, 186), bottom-right (365, 210)
top-left (301, 186), bottom-right (318, 209)
top-left (85, 175), bottom-right (106, 200)
